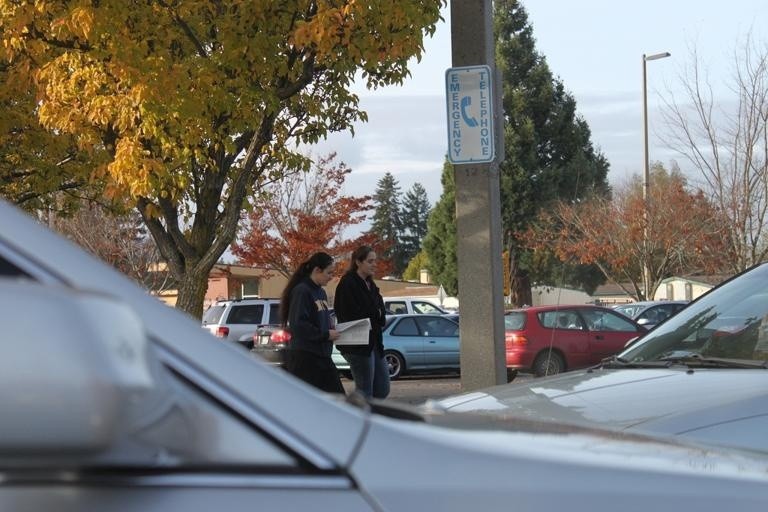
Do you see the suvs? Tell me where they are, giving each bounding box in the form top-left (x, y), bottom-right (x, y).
top-left (200, 297), bottom-right (281, 347)
top-left (380, 297), bottom-right (456, 314)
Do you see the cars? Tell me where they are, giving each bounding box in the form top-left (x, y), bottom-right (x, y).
top-left (504, 307), bottom-right (649, 379)
top-left (421, 263), bottom-right (766, 455)
top-left (594, 300), bottom-right (692, 332)
top-left (1, 197), bottom-right (767, 507)
top-left (252, 309), bottom-right (332, 365)
top-left (332, 313), bottom-right (460, 377)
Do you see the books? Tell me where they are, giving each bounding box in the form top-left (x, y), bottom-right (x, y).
top-left (333, 318), bottom-right (373, 346)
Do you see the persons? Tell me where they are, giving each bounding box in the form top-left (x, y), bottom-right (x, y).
top-left (278, 252), bottom-right (347, 397)
top-left (558, 316), bottom-right (570, 330)
top-left (333, 245), bottom-right (392, 398)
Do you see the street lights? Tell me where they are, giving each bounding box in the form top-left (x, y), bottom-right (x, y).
top-left (639, 52), bottom-right (671, 301)
